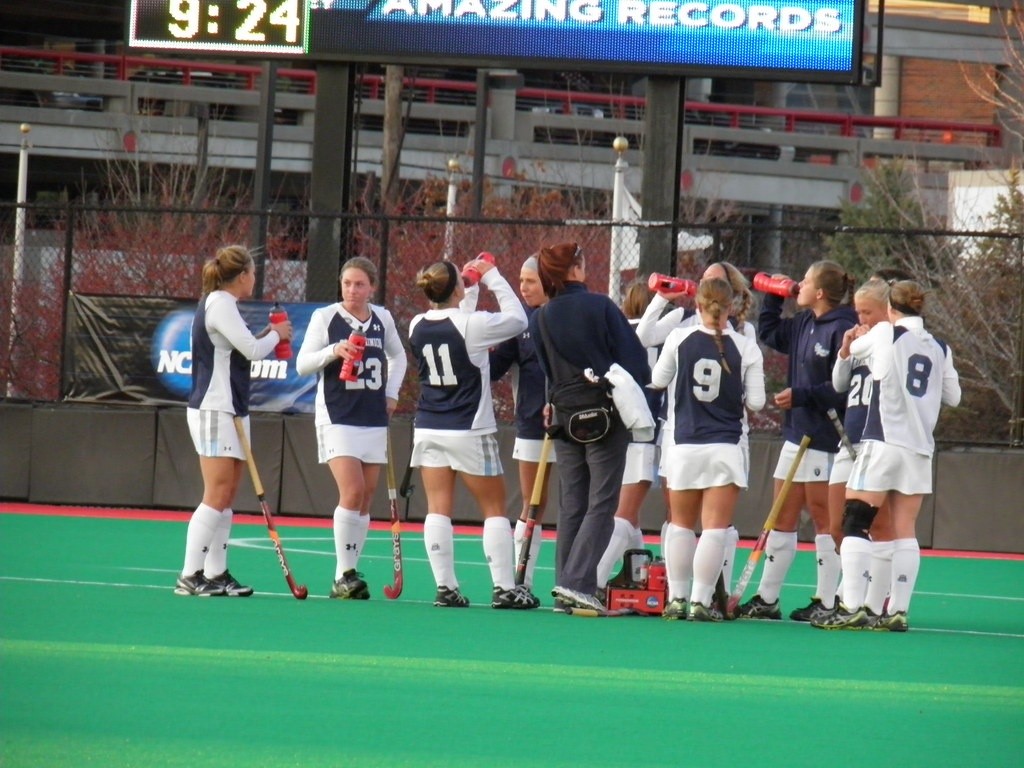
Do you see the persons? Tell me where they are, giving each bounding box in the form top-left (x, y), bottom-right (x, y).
top-left (740, 260), bottom-right (859, 623)
top-left (173, 245), bottom-right (293, 597)
top-left (490, 243), bottom-right (767, 617)
top-left (410, 259), bottom-right (540, 609)
top-left (809, 268), bottom-right (960, 632)
top-left (295, 257), bottom-right (407, 601)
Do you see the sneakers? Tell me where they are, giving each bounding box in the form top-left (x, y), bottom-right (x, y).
top-left (875, 611), bottom-right (909, 631)
top-left (686, 598), bottom-right (726, 622)
top-left (549, 586), bottom-right (610, 612)
top-left (491, 584), bottom-right (540, 609)
top-left (330, 564), bottom-right (372, 600)
top-left (789, 596), bottom-right (840, 622)
top-left (175, 567), bottom-right (226, 598)
top-left (738, 593), bottom-right (783, 620)
top-left (659, 598), bottom-right (692, 621)
top-left (201, 568), bottom-right (255, 599)
top-left (810, 602), bottom-right (870, 629)
top-left (432, 583), bottom-right (472, 608)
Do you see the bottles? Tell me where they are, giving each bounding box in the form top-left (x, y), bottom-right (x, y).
top-left (648, 272), bottom-right (697, 298)
top-left (648, 556), bottom-right (665, 591)
top-left (269, 302), bottom-right (292, 359)
top-left (753, 272), bottom-right (799, 298)
top-left (460, 251), bottom-right (495, 287)
top-left (339, 325), bottom-right (367, 382)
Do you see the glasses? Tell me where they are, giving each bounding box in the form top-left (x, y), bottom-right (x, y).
top-left (571, 242), bottom-right (583, 261)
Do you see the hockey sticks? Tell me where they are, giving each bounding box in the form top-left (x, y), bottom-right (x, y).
top-left (232, 414), bottom-right (310, 600)
top-left (825, 407), bottom-right (859, 463)
top-left (383, 419), bottom-right (404, 600)
top-left (716, 569), bottom-right (743, 621)
top-left (714, 430), bottom-right (813, 618)
top-left (514, 432), bottom-right (551, 586)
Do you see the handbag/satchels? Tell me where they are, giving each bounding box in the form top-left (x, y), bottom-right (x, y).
top-left (548, 373), bottom-right (617, 446)
